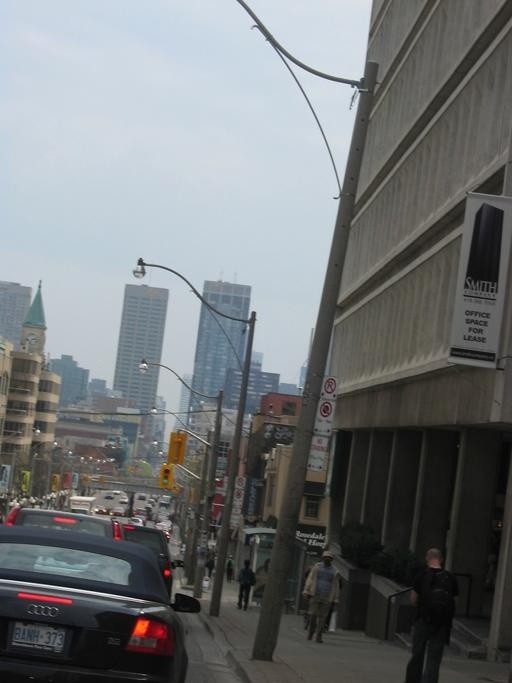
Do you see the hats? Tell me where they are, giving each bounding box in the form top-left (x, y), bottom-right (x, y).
top-left (320, 551), bottom-right (334, 561)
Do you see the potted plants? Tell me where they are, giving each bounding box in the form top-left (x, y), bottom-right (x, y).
top-left (333, 522), bottom-right (420, 642)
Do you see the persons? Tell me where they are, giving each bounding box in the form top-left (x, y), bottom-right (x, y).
top-left (405, 549), bottom-right (459, 683)
top-left (237, 560), bottom-right (252, 611)
top-left (0, 490), bottom-right (69, 523)
top-left (303, 550), bottom-right (342, 643)
top-left (226, 555), bottom-right (234, 584)
top-left (253, 559), bottom-right (272, 598)
top-left (205, 545), bottom-right (215, 578)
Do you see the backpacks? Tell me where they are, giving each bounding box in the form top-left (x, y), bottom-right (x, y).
top-left (241, 568), bottom-right (256, 586)
top-left (421, 570), bottom-right (452, 623)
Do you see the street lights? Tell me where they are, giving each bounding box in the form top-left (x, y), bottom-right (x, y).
top-left (129, 257), bottom-right (258, 620)
top-left (138, 359), bottom-right (225, 599)
